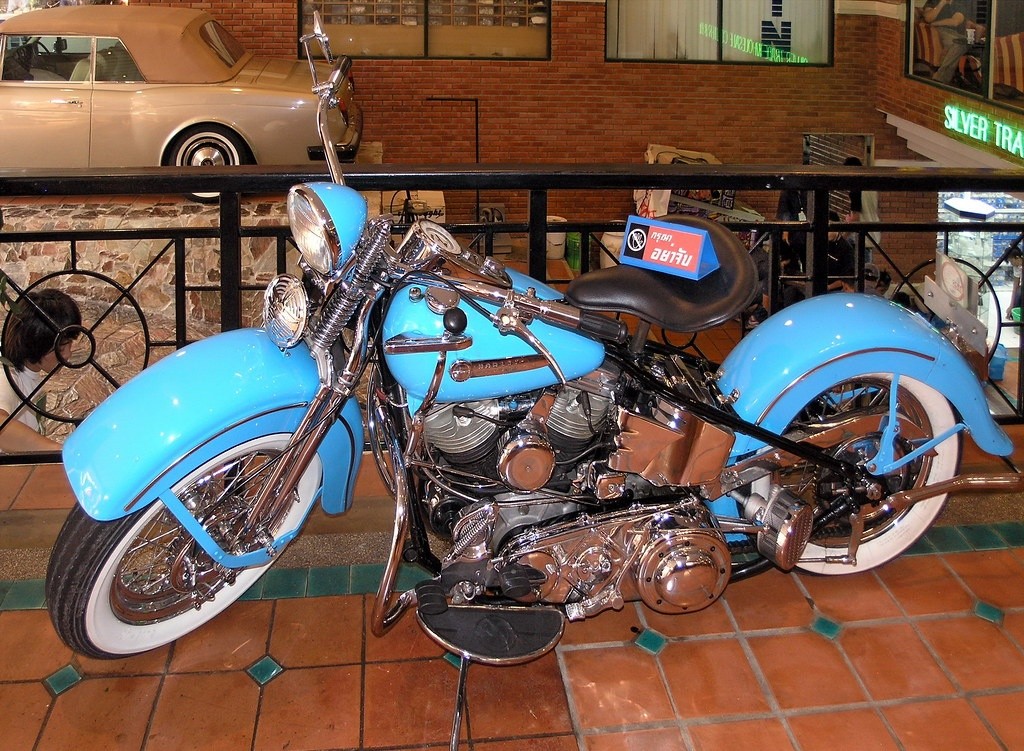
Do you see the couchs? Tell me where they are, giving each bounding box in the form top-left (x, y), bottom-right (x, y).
top-left (914, 6), bottom-right (988, 67)
top-left (993, 32), bottom-right (1024, 93)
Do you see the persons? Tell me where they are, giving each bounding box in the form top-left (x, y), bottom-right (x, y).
top-left (0, 288), bottom-right (83, 452)
top-left (841, 156), bottom-right (881, 264)
top-left (922, 0), bottom-right (991, 85)
top-left (1003, 244), bottom-right (1024, 323)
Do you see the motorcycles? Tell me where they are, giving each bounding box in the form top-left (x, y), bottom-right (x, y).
top-left (45, 9), bottom-right (1012, 668)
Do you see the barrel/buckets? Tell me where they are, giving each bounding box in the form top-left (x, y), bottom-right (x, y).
top-left (1011, 307), bottom-right (1020, 335)
top-left (599, 220), bottom-right (628, 268)
top-left (566, 233), bottom-right (592, 271)
top-left (547, 216), bottom-right (568, 259)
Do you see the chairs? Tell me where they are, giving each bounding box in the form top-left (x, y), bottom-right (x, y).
top-left (69, 49), bottom-right (118, 81)
top-left (29, 41), bottom-right (130, 81)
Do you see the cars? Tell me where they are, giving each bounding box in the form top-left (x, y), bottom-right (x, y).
top-left (0, 4), bottom-right (364, 202)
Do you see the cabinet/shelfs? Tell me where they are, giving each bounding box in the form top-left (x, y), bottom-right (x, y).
top-left (943, 198), bottom-right (995, 339)
top-left (428, 0), bottom-right (548, 58)
top-left (302, 0), bottom-right (424, 57)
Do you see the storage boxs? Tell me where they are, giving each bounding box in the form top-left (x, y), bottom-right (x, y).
top-left (644, 143), bottom-right (736, 210)
top-left (382, 191), bottom-right (446, 248)
top-left (633, 190), bottom-right (766, 253)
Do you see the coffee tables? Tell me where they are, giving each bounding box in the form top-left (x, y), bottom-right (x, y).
top-left (952, 37), bottom-right (988, 81)
